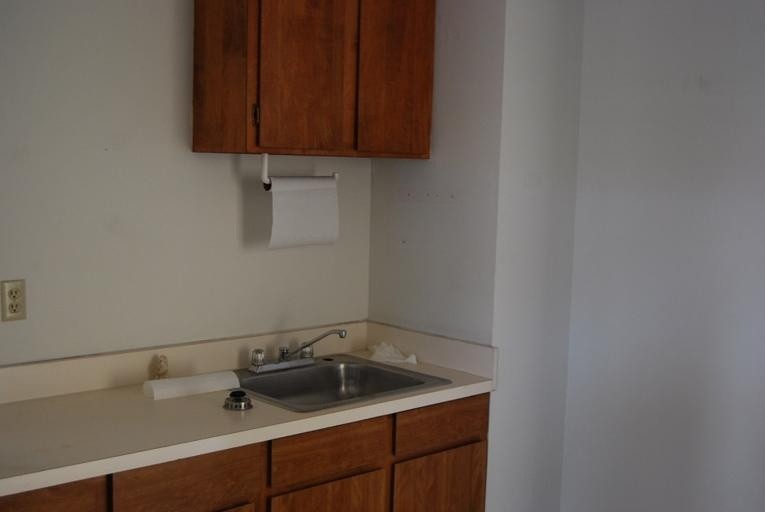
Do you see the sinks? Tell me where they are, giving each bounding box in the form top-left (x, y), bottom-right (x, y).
top-left (235, 352), bottom-right (452, 413)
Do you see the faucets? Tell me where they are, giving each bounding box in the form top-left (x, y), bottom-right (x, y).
top-left (279, 328), bottom-right (347, 361)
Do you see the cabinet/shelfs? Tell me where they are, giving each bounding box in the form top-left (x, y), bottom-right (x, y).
top-left (190, 0), bottom-right (437, 162)
top-left (267, 391), bottom-right (490, 512)
top-left (0, 438), bottom-right (267, 512)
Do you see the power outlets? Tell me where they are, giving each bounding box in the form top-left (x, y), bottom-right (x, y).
top-left (0, 278), bottom-right (27, 320)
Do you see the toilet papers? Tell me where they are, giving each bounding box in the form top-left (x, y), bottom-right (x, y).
top-left (262, 175), bottom-right (339, 251)
